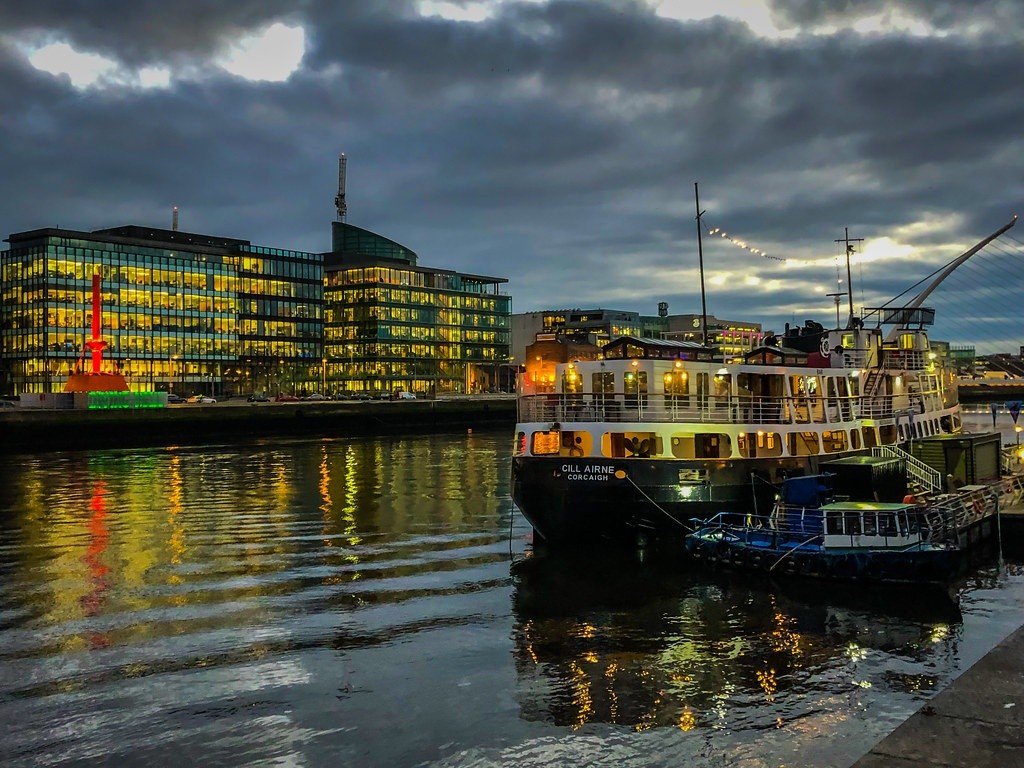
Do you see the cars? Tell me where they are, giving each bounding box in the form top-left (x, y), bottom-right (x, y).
top-left (188, 394), bottom-right (217, 404)
top-left (300, 393), bottom-right (324, 402)
top-left (168, 394), bottom-right (187, 404)
top-left (278, 395), bottom-right (299, 401)
top-left (247, 395), bottom-right (270, 403)
top-left (326, 394), bottom-right (349, 401)
top-left (350, 392), bottom-right (375, 401)
top-left (375, 392), bottom-right (394, 401)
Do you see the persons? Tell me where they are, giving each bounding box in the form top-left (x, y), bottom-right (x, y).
top-left (903, 488), bottom-right (917, 504)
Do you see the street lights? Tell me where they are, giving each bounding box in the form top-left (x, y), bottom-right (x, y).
top-left (321, 358), bottom-right (327, 398)
top-left (169, 355), bottom-right (179, 393)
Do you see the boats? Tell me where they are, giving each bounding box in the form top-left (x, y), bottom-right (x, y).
top-left (509, 180), bottom-right (964, 552)
top-left (685, 510), bottom-right (964, 577)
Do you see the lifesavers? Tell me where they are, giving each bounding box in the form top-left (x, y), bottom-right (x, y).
top-left (973, 499), bottom-right (983, 514)
top-left (569, 444), bottom-right (585, 457)
top-left (1003, 483), bottom-right (1013, 494)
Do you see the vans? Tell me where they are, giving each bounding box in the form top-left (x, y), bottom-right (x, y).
top-left (399, 392), bottom-right (417, 400)
